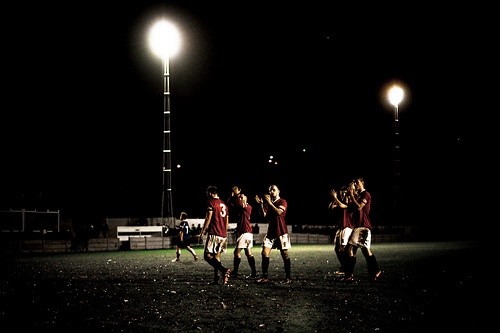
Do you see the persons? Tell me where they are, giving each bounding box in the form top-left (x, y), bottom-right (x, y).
top-left (190, 223), bottom-right (201, 233)
top-left (170, 212), bottom-right (199, 263)
top-left (328, 177), bottom-right (383, 283)
top-left (254, 182), bottom-right (293, 285)
top-left (200, 186), bottom-right (231, 286)
top-left (226, 184), bottom-right (257, 280)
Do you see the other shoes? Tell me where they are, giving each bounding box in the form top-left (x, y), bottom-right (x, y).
top-left (246, 273), bottom-right (257, 279)
top-left (340, 276), bottom-right (353, 281)
top-left (222, 268), bottom-right (232, 285)
top-left (227, 273), bottom-right (237, 279)
top-left (206, 281), bottom-right (219, 285)
top-left (282, 278), bottom-right (293, 284)
top-left (373, 270), bottom-right (382, 281)
top-left (256, 277), bottom-right (269, 283)
top-left (333, 271), bottom-right (345, 275)
